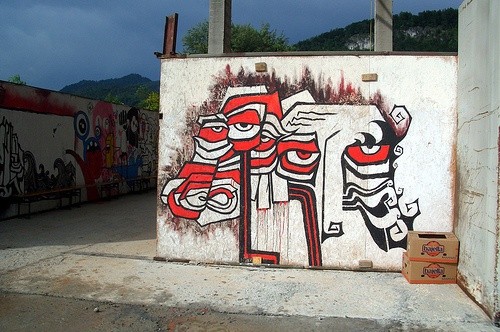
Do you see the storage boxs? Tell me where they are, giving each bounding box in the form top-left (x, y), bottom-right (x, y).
top-left (402, 252), bottom-right (456, 284)
top-left (407, 231), bottom-right (459, 263)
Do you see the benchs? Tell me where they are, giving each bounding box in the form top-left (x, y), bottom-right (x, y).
top-left (100, 177), bottom-right (150, 200)
top-left (15, 187), bottom-right (81, 217)
